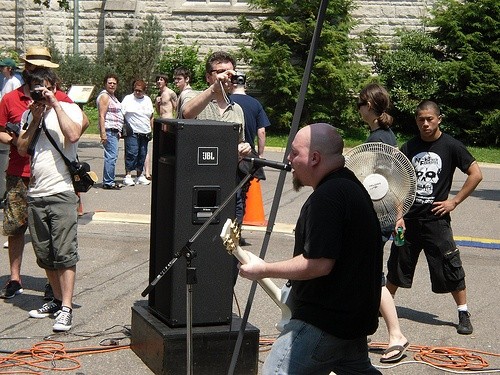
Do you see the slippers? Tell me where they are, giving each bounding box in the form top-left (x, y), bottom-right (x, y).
top-left (380, 341), bottom-right (411, 362)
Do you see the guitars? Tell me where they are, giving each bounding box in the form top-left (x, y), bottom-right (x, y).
top-left (220, 218), bottom-right (293, 332)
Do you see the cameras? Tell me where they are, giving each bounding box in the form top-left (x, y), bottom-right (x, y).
top-left (5, 121), bottom-right (21, 137)
top-left (30, 84), bottom-right (47, 101)
top-left (231, 73), bottom-right (246, 84)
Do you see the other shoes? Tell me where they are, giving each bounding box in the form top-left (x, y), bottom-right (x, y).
top-left (240, 237), bottom-right (245, 246)
top-left (103, 180), bottom-right (124, 189)
top-left (145, 174), bottom-right (152, 180)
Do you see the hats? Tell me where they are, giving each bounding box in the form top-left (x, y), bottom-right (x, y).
top-left (20, 45), bottom-right (60, 68)
top-left (0, 57), bottom-right (16, 70)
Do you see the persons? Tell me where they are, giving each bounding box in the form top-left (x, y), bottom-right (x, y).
top-left (173, 66), bottom-right (194, 120)
top-left (355, 82), bottom-right (411, 362)
top-left (237, 121), bottom-right (384, 375)
top-left (0, 44), bottom-right (75, 299)
top-left (97, 73), bottom-right (125, 190)
top-left (154, 73), bottom-right (178, 118)
top-left (0, 57), bottom-right (25, 100)
top-left (177, 50), bottom-right (253, 158)
top-left (16, 65), bottom-right (84, 332)
top-left (120, 80), bottom-right (153, 186)
top-left (226, 69), bottom-right (272, 246)
top-left (385, 98), bottom-right (484, 336)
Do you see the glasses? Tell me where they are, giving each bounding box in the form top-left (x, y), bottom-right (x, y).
top-left (212, 69), bottom-right (226, 74)
top-left (357, 101), bottom-right (368, 110)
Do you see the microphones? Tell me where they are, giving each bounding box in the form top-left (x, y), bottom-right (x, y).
top-left (244, 156), bottom-right (292, 172)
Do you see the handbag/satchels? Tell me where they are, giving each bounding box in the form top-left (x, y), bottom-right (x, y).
top-left (69, 161), bottom-right (98, 193)
top-left (120, 119), bottom-right (133, 138)
top-left (137, 132), bottom-right (153, 141)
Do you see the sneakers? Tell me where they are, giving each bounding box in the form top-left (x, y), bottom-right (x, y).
top-left (52, 309), bottom-right (72, 331)
top-left (43, 282), bottom-right (54, 300)
top-left (0, 279), bottom-right (23, 298)
top-left (135, 174), bottom-right (151, 185)
top-left (457, 311), bottom-right (473, 334)
top-left (123, 174), bottom-right (134, 186)
top-left (29, 298), bottom-right (61, 318)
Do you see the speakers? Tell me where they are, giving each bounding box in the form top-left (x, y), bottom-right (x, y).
top-left (149, 118), bottom-right (241, 327)
